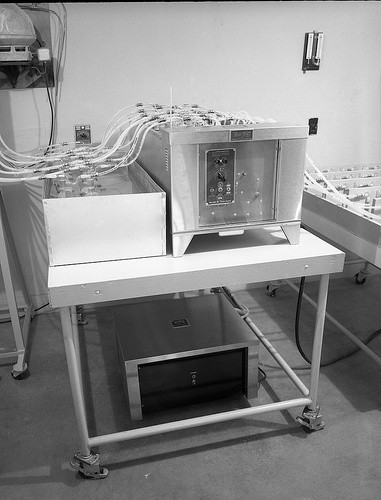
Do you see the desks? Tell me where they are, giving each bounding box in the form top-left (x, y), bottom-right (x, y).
top-left (45, 226), bottom-right (345, 478)
